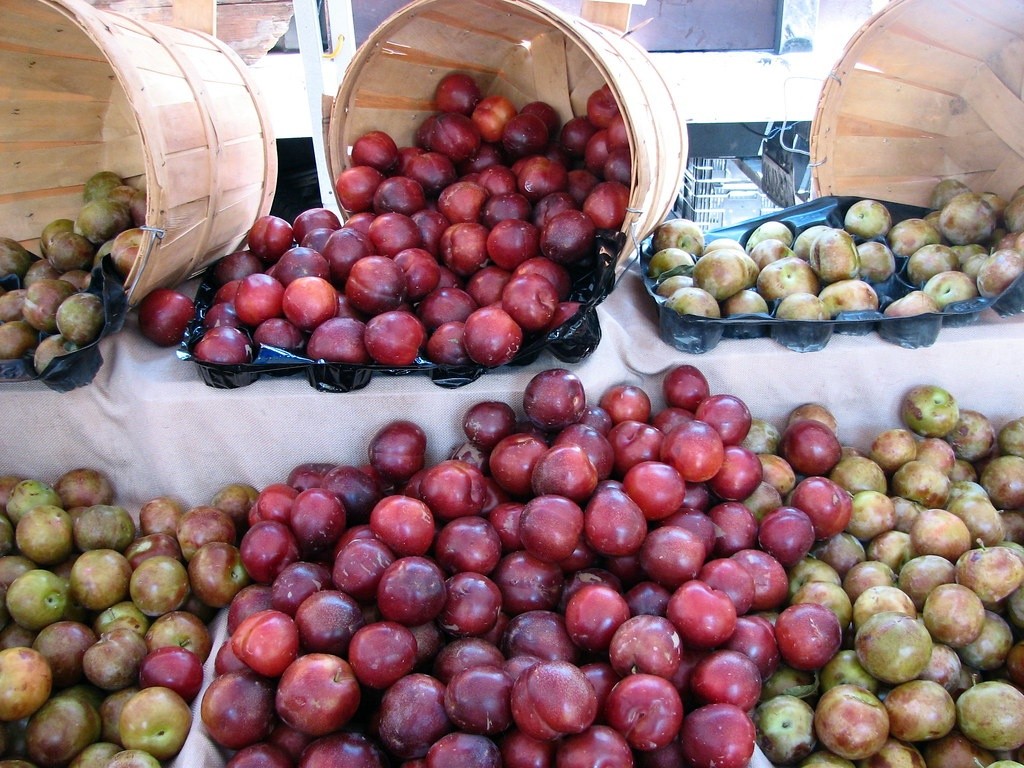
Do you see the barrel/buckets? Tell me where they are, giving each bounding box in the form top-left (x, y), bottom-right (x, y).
top-left (3, 1), bottom-right (278, 339)
top-left (808, 1), bottom-right (1024, 209)
top-left (322, 1), bottom-right (687, 296)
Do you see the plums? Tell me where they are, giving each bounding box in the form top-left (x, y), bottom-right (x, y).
top-left (0, 71), bottom-right (1024, 768)
